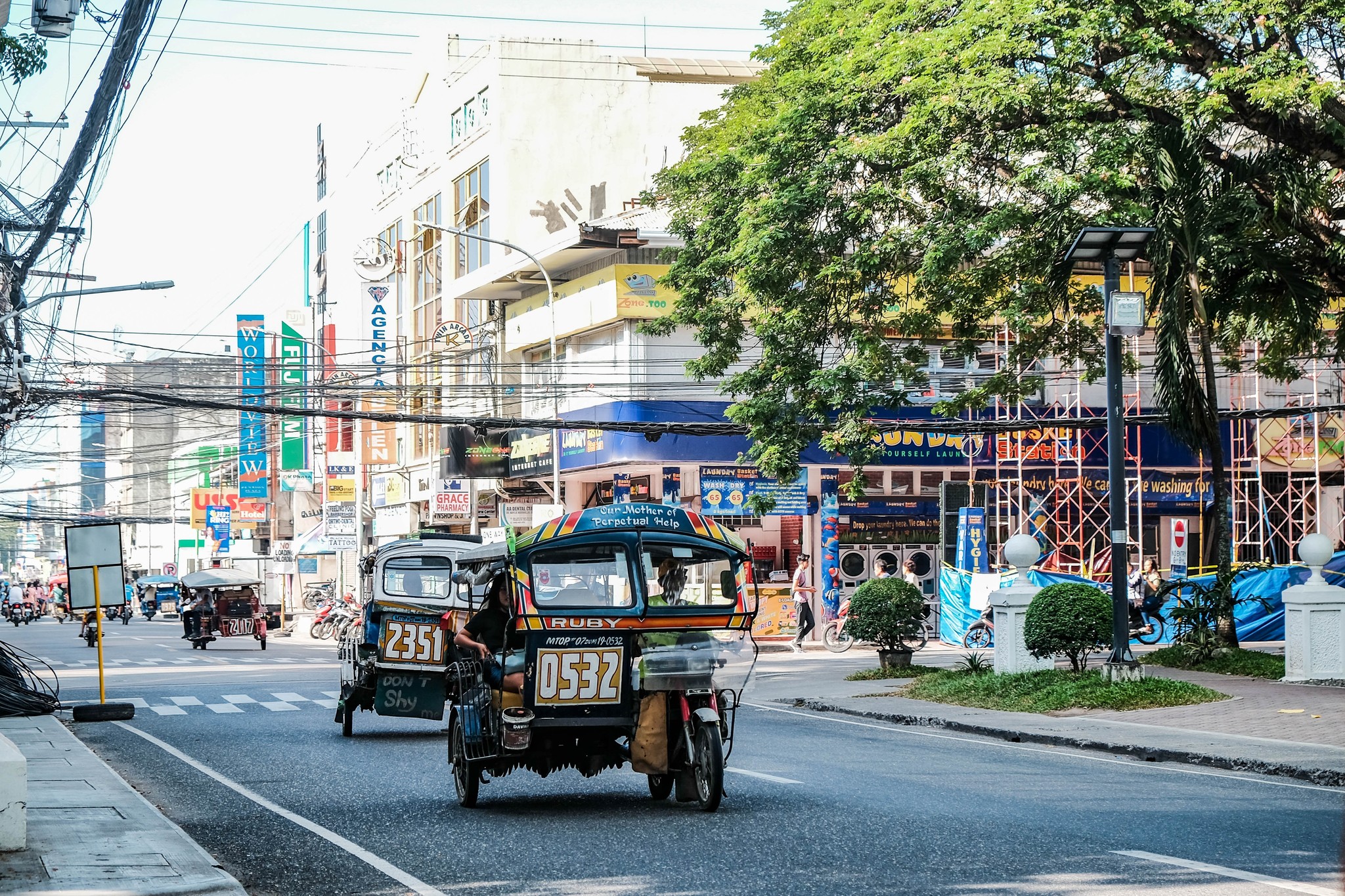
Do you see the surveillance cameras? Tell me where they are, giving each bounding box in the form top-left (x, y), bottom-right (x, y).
top-left (19, 353), bottom-right (30, 363)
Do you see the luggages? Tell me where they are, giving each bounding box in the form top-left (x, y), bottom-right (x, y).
top-left (227, 600), bottom-right (252, 618)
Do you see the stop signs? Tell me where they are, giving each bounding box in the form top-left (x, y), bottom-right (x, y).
top-left (1174, 522), bottom-right (1185, 547)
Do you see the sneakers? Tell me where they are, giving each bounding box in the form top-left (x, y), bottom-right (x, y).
top-left (788, 638), bottom-right (806, 654)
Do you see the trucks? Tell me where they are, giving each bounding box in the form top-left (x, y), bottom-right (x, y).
top-left (219, 556), bottom-right (284, 628)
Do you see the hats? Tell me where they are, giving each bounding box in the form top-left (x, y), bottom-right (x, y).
top-left (4, 581), bottom-right (9, 586)
top-left (658, 558), bottom-right (688, 578)
top-left (13, 581), bottom-right (19, 585)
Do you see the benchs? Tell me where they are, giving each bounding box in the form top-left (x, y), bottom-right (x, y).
top-left (439, 610), bottom-right (524, 731)
top-left (217, 587), bottom-right (258, 615)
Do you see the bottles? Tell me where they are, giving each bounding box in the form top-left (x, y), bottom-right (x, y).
top-left (752, 559), bottom-right (773, 579)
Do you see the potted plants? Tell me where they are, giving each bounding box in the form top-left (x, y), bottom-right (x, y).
top-left (844, 577), bottom-right (928, 668)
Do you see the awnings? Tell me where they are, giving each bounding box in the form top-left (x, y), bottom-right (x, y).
top-left (291, 520), bottom-right (336, 555)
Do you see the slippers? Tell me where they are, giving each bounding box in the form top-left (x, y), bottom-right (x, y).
top-left (1139, 627), bottom-right (1152, 634)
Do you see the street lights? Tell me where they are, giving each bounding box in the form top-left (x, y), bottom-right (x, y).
top-left (417, 220), bottom-right (561, 515)
top-left (59, 489), bottom-right (94, 516)
top-left (1064, 224), bottom-right (1162, 661)
top-left (243, 327), bottom-right (343, 597)
top-left (91, 443), bottom-right (151, 577)
top-left (78, 474), bottom-right (117, 516)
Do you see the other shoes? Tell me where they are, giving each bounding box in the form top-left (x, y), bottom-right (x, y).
top-left (21, 616), bottom-right (26, 621)
top-left (190, 632), bottom-right (200, 637)
top-left (6, 618), bottom-right (11, 622)
top-left (40, 612), bottom-right (46, 615)
top-left (141, 615), bottom-right (146, 619)
top-left (33, 615), bottom-right (39, 617)
top-left (79, 633), bottom-right (83, 637)
top-left (182, 634), bottom-right (190, 639)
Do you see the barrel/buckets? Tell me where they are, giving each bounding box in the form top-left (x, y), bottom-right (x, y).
top-left (356, 643), bottom-right (380, 673)
top-left (501, 706), bottom-right (535, 750)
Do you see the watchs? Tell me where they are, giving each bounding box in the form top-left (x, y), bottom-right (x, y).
top-left (194, 603), bottom-right (197, 606)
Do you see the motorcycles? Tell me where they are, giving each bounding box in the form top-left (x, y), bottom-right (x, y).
top-left (3, 598), bottom-right (40, 628)
top-left (104, 606), bottom-right (117, 619)
top-left (56, 603), bottom-right (66, 623)
top-left (136, 575), bottom-right (181, 620)
top-left (179, 568), bottom-right (268, 649)
top-left (302, 579), bottom-right (362, 640)
top-left (1129, 605), bottom-right (1170, 644)
top-left (335, 530), bottom-right (499, 737)
top-left (821, 596), bottom-right (934, 652)
top-left (79, 612), bottom-right (105, 647)
top-left (444, 498), bottom-right (759, 810)
top-left (962, 597), bottom-right (996, 649)
top-left (119, 603), bottom-right (131, 624)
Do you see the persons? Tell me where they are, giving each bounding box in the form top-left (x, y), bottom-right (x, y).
top-left (403, 571), bottom-right (423, 596)
top-left (0, 577), bottom-right (157, 622)
top-left (1127, 559), bottom-right (1162, 633)
top-left (453, 571), bottom-right (525, 708)
top-left (459, 586), bottom-right (491, 602)
top-left (181, 587), bottom-right (216, 639)
top-left (79, 608), bottom-right (103, 638)
top-left (631, 558), bottom-right (741, 746)
top-left (788, 553), bottom-right (817, 653)
top-left (566, 564), bottom-right (607, 607)
top-left (756, 570), bottom-right (767, 584)
top-left (204, 527), bottom-right (226, 552)
top-left (874, 559), bottom-right (919, 589)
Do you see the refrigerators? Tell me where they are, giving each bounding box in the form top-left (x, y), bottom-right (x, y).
top-left (746, 546), bottom-right (776, 583)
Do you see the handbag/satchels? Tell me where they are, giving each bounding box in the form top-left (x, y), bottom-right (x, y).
top-left (789, 568), bottom-right (806, 599)
top-left (59, 595), bottom-right (66, 603)
